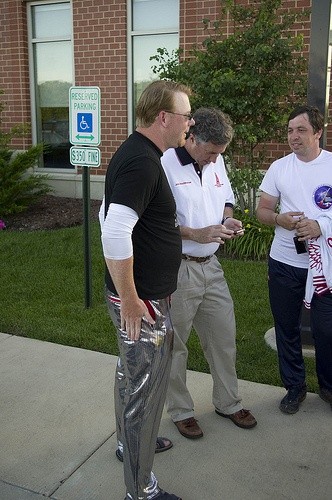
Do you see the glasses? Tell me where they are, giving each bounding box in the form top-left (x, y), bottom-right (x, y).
top-left (155, 111), bottom-right (193, 122)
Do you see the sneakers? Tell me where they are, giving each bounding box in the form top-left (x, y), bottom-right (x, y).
top-left (215, 409), bottom-right (257, 428)
top-left (172, 416), bottom-right (203, 439)
top-left (320, 390), bottom-right (332, 411)
top-left (279, 385), bottom-right (307, 413)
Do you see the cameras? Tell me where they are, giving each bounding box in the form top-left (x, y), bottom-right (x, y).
top-left (294, 236), bottom-right (307, 254)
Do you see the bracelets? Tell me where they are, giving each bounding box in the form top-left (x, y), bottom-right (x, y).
top-left (275, 215), bottom-right (280, 226)
top-left (221, 217), bottom-right (232, 225)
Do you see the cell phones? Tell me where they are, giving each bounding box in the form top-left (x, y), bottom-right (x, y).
top-left (233, 229), bottom-right (245, 234)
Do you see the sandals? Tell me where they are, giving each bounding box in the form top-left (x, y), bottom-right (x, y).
top-left (125, 490), bottom-right (184, 500)
top-left (115, 437), bottom-right (173, 461)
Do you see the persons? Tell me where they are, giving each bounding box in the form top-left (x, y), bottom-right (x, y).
top-left (159, 107), bottom-right (258, 438)
top-left (98, 80), bottom-right (196, 500)
top-left (256, 105), bottom-right (332, 414)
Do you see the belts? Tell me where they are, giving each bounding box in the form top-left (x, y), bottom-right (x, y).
top-left (182, 254), bottom-right (212, 263)
